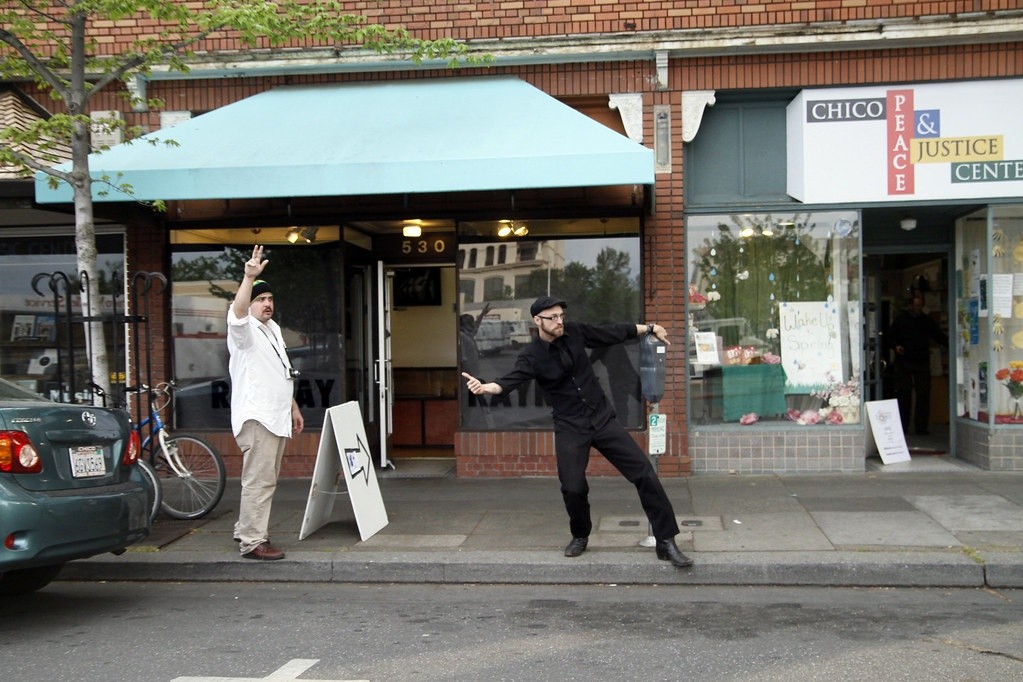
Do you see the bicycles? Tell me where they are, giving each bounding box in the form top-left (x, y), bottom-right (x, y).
top-left (83, 384), bottom-right (226, 521)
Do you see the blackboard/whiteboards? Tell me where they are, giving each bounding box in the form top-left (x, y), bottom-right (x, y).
top-left (779, 301), bottom-right (844, 387)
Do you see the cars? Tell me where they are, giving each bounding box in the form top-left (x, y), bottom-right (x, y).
top-left (172, 341), bottom-right (335, 429)
top-left (1, 377), bottom-right (147, 597)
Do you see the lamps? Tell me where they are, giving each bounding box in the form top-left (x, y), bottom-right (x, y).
top-left (286, 227), bottom-right (320, 243)
top-left (403, 223), bottom-right (422, 237)
top-left (497, 221), bottom-right (526, 237)
top-left (901, 219), bottom-right (918, 230)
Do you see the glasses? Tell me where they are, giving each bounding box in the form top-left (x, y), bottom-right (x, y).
top-left (538, 313), bottom-right (566, 321)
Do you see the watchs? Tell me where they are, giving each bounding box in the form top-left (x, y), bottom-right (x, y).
top-left (649, 324), bottom-right (654, 332)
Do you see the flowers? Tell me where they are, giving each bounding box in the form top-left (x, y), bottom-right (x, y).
top-left (995, 361), bottom-right (1023, 396)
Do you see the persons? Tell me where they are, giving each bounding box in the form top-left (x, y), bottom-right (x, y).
top-left (460, 303), bottom-right (491, 407)
top-left (886, 297), bottom-right (949, 435)
top-left (226, 244), bottom-right (304, 561)
top-left (588, 318), bottom-right (642, 427)
top-left (461, 296), bottom-right (693, 568)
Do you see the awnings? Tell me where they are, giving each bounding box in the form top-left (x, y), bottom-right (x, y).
top-left (34, 74), bottom-right (654, 205)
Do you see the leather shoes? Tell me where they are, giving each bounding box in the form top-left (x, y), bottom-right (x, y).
top-left (242, 543), bottom-right (285, 560)
top-left (234, 537), bottom-right (270, 545)
top-left (656, 536), bottom-right (693, 566)
top-left (565, 536), bottom-right (588, 556)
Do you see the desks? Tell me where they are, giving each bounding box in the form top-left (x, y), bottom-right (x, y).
top-left (699, 363), bottom-right (786, 425)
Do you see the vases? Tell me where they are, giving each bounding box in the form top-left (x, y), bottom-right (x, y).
top-left (1007, 393), bottom-right (1023, 424)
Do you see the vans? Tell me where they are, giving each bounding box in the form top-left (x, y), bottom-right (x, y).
top-left (472, 320), bottom-right (533, 357)
top-left (691, 318), bottom-right (756, 370)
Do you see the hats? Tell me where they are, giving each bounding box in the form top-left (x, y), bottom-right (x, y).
top-left (250, 280), bottom-right (273, 306)
top-left (531, 296), bottom-right (567, 318)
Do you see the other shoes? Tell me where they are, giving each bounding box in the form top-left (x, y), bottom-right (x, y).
top-left (916, 430), bottom-right (930, 436)
top-left (904, 429), bottom-right (908, 435)
